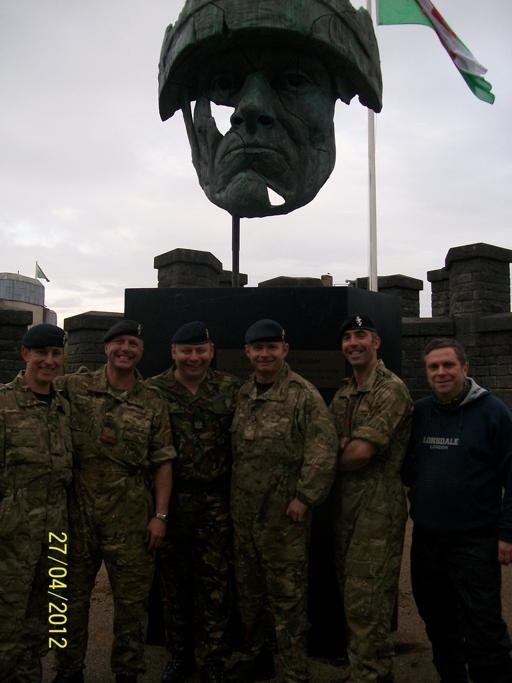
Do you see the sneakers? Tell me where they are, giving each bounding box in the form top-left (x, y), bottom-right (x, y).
top-left (223, 650), bottom-right (278, 682)
top-left (116, 673), bottom-right (137, 683)
top-left (161, 647), bottom-right (199, 683)
top-left (51, 670), bottom-right (84, 682)
top-left (199, 657), bottom-right (226, 683)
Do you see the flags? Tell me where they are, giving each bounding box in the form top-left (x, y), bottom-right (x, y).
top-left (376, 0), bottom-right (495, 105)
top-left (36, 264), bottom-right (50, 282)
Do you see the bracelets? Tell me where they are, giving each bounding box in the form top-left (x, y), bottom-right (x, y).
top-left (153, 512), bottom-right (171, 522)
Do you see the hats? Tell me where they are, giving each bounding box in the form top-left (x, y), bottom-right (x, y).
top-left (170, 319), bottom-right (211, 348)
top-left (103, 318), bottom-right (147, 346)
top-left (338, 313), bottom-right (379, 336)
top-left (19, 322), bottom-right (68, 352)
top-left (244, 316), bottom-right (287, 344)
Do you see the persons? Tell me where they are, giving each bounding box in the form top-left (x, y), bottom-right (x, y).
top-left (156, 0), bottom-right (383, 219)
top-left (401, 338), bottom-right (511, 683)
top-left (228, 319), bottom-right (340, 682)
top-left (141, 320), bottom-right (244, 682)
top-left (320, 314), bottom-right (414, 682)
top-left (0, 324), bottom-right (77, 682)
top-left (53, 319), bottom-right (178, 683)
